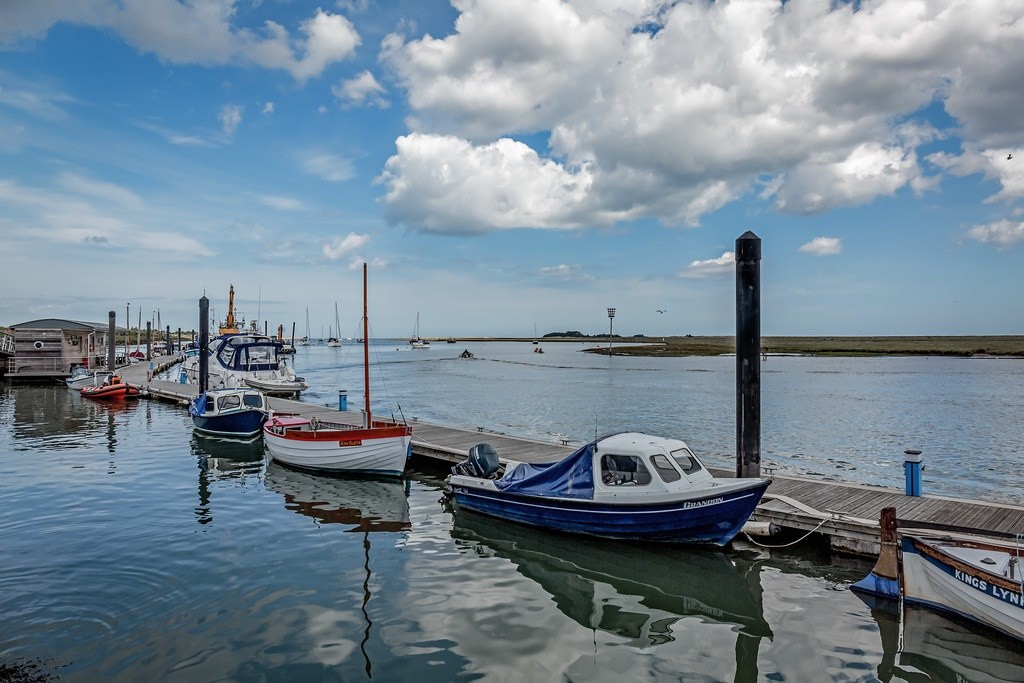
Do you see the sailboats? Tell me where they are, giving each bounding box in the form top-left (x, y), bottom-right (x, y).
top-left (318, 325), bottom-right (326, 344)
top-left (297, 307), bottom-right (312, 345)
top-left (357, 321), bottom-right (364, 343)
top-left (532, 322), bottom-right (539, 344)
top-left (408, 312), bottom-right (430, 349)
top-left (334, 301), bottom-right (343, 342)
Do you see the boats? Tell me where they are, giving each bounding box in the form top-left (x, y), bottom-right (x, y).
top-left (896, 598), bottom-right (1024, 683)
top-left (327, 336), bottom-right (342, 348)
top-left (439, 430), bottom-right (775, 548)
top-left (533, 347), bottom-right (545, 353)
top-left (443, 508), bottom-right (775, 649)
top-left (458, 350), bottom-right (474, 360)
top-left (263, 407), bottom-right (412, 475)
top-left (79, 373), bottom-right (138, 398)
top-left (895, 527), bottom-right (1024, 644)
top-left (447, 337), bottom-right (455, 343)
top-left (188, 386), bottom-right (271, 445)
top-left (126, 284), bottom-right (311, 397)
top-left (66, 372), bottom-right (95, 390)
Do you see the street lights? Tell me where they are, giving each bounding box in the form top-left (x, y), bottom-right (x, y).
top-left (606, 307), bottom-right (616, 356)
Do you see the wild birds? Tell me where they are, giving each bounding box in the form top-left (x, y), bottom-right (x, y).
top-left (597, 344), bottom-right (600, 347)
top-left (1006, 153), bottom-right (1013, 160)
top-left (656, 309), bottom-right (668, 314)
